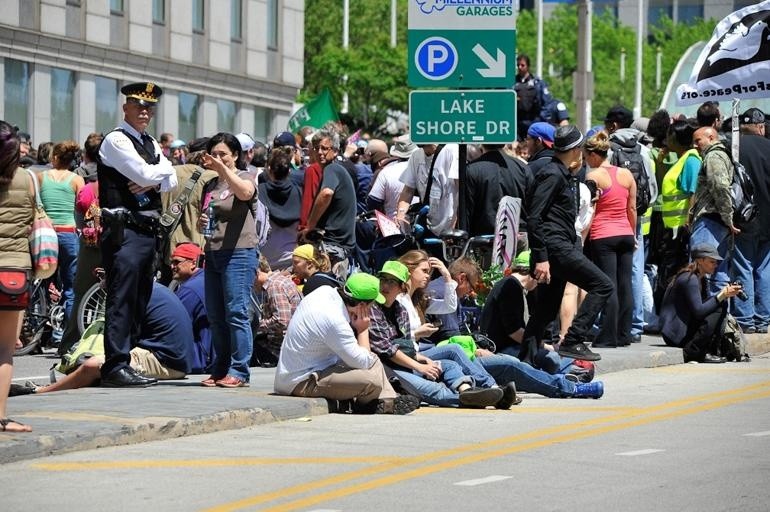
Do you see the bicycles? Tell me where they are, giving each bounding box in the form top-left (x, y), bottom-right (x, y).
top-left (423, 227), bottom-right (496, 348)
top-left (15, 278), bottom-right (113, 358)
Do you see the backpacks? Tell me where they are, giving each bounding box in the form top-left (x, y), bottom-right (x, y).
top-left (237, 170), bottom-right (271, 250)
top-left (609, 136), bottom-right (651, 216)
top-left (50, 317), bottom-right (104, 382)
top-left (712, 147), bottom-right (759, 228)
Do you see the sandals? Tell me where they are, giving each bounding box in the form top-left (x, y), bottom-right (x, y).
top-left (0, 419), bottom-right (32, 432)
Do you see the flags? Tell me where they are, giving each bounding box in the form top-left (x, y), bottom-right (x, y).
top-left (284, 84), bottom-right (345, 133)
top-left (676, 0), bottom-right (770, 106)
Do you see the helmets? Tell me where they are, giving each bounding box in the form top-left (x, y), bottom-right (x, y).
top-left (171, 139), bottom-right (185, 148)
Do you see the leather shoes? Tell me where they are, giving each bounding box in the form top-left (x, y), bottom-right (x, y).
top-left (102, 366), bottom-right (158, 388)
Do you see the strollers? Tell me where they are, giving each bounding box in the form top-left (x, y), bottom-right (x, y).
top-left (346, 206), bottom-right (432, 270)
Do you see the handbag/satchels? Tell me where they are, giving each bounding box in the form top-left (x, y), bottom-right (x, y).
top-left (99, 219), bottom-right (123, 257)
top-left (26, 169), bottom-right (59, 279)
top-left (159, 171), bottom-right (201, 238)
top-left (0, 269), bottom-right (30, 310)
top-left (406, 202), bottom-right (431, 239)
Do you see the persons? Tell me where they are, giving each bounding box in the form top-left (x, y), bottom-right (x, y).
top-left (27, 244), bottom-right (193, 394)
top-left (98, 81), bottom-right (179, 390)
top-left (509, 56), bottom-right (552, 136)
top-left (0, 119), bottom-right (63, 432)
top-left (158, 105), bottom-right (770, 414)
top-left (16, 132), bottom-right (105, 358)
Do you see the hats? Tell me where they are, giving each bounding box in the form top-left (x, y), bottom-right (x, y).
top-left (292, 244), bottom-right (320, 261)
top-left (554, 125), bottom-right (583, 151)
top-left (371, 151), bottom-right (389, 173)
top-left (741, 108), bottom-right (767, 124)
top-left (528, 122), bottom-right (556, 148)
top-left (379, 260), bottom-right (410, 282)
top-left (390, 134), bottom-right (418, 159)
top-left (344, 273), bottom-right (386, 304)
top-left (598, 104), bottom-right (633, 125)
top-left (514, 250), bottom-right (531, 267)
top-left (236, 133), bottom-right (255, 152)
top-left (274, 132), bottom-right (301, 149)
top-left (189, 137), bottom-right (210, 151)
top-left (172, 244), bottom-right (201, 260)
top-left (691, 243), bottom-right (724, 260)
top-left (121, 82), bottom-right (162, 106)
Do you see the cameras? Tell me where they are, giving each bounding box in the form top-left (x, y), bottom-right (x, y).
top-left (731, 280), bottom-right (749, 302)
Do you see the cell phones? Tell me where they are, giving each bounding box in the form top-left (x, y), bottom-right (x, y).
top-left (433, 318), bottom-right (443, 328)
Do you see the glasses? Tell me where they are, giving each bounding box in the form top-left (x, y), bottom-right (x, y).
top-left (461, 271), bottom-right (480, 293)
top-left (313, 146), bottom-right (332, 151)
top-left (169, 260), bottom-right (184, 265)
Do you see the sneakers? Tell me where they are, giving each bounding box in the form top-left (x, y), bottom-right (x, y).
top-left (216, 374), bottom-right (250, 386)
top-left (201, 376), bottom-right (218, 387)
top-left (25, 380), bottom-right (38, 393)
